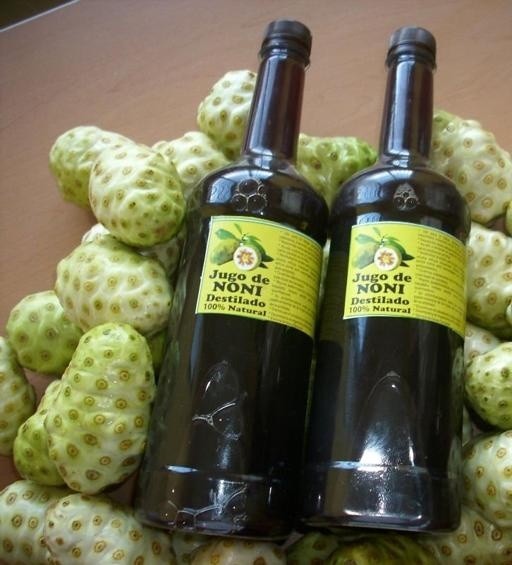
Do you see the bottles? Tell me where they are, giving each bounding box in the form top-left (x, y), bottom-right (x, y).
top-left (300, 24), bottom-right (479, 539)
top-left (130, 14), bottom-right (311, 545)
